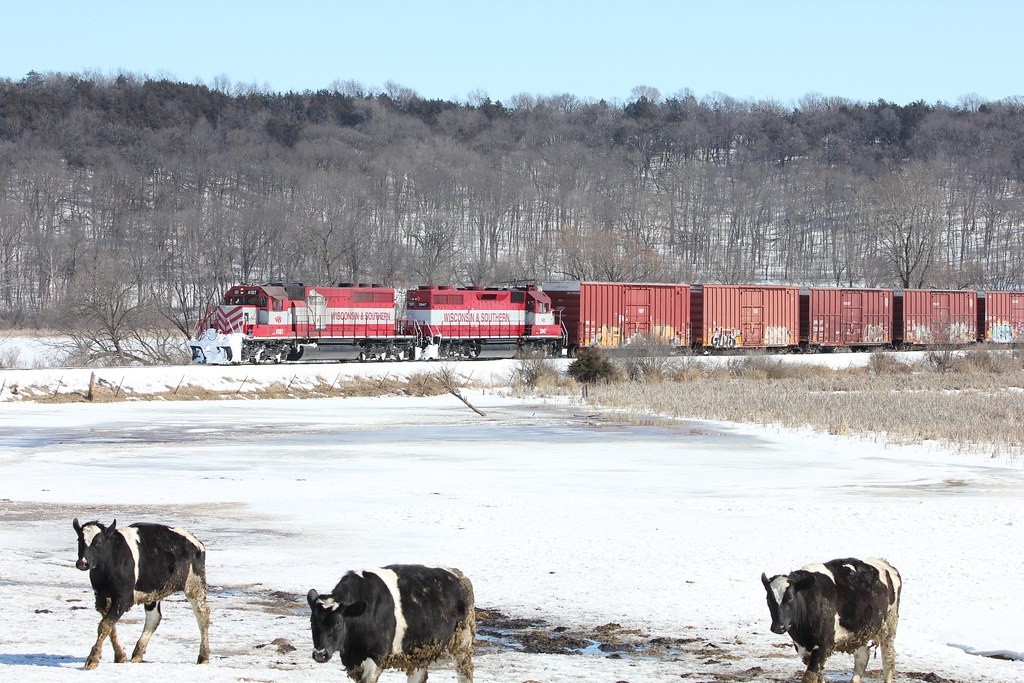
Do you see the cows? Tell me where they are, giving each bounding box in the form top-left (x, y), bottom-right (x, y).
top-left (761, 556), bottom-right (902, 683)
top-left (74, 518), bottom-right (211, 671)
top-left (307, 564), bottom-right (476, 683)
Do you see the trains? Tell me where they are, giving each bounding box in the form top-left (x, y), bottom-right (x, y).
top-left (189, 277), bottom-right (1024, 367)
